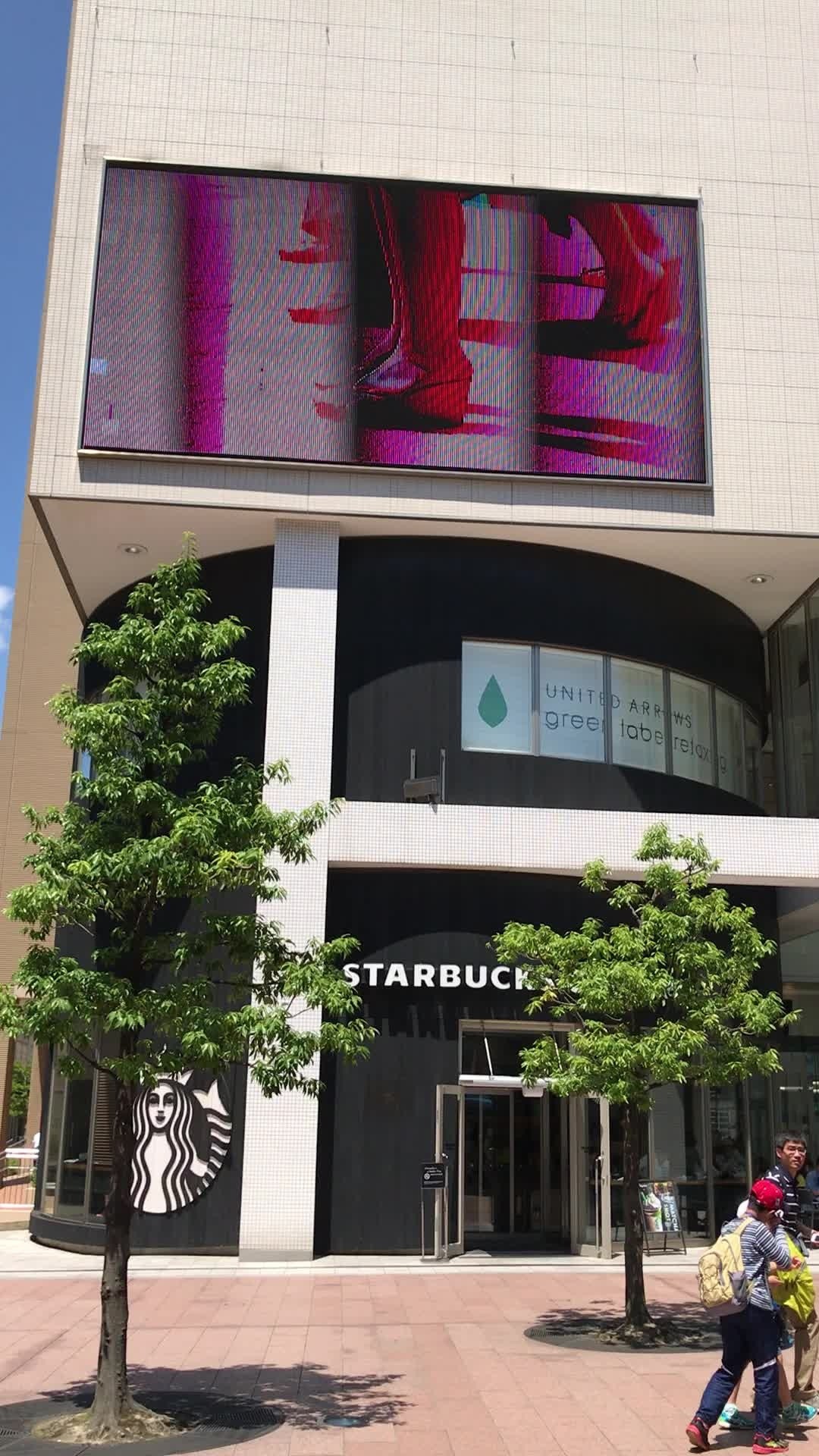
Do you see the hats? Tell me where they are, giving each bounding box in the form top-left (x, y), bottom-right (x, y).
top-left (749, 1180), bottom-right (784, 1209)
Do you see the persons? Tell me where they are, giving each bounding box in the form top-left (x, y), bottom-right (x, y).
top-left (685, 1130), bottom-right (819, 1202)
top-left (756, 1130), bottom-right (819, 1414)
top-left (686, 1181), bottom-right (788, 1454)
top-left (717, 1199), bottom-right (816, 1431)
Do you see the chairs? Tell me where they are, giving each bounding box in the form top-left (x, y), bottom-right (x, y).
top-left (798, 1189), bottom-right (819, 1250)
top-left (676, 1183), bottom-right (709, 1237)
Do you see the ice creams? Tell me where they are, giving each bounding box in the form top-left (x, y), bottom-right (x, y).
top-left (642, 1191), bottom-right (663, 1232)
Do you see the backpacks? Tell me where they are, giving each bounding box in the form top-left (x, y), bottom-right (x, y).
top-left (696, 1218), bottom-right (754, 1316)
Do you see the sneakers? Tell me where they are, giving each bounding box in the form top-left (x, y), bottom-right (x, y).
top-left (783, 1401), bottom-right (817, 1428)
top-left (752, 1432), bottom-right (789, 1454)
top-left (752, 1404), bottom-right (782, 1420)
top-left (686, 1417), bottom-right (710, 1450)
top-left (718, 1403), bottom-right (755, 1429)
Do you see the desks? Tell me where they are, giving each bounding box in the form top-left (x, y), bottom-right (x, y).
top-left (590, 1179), bottom-right (747, 1231)
